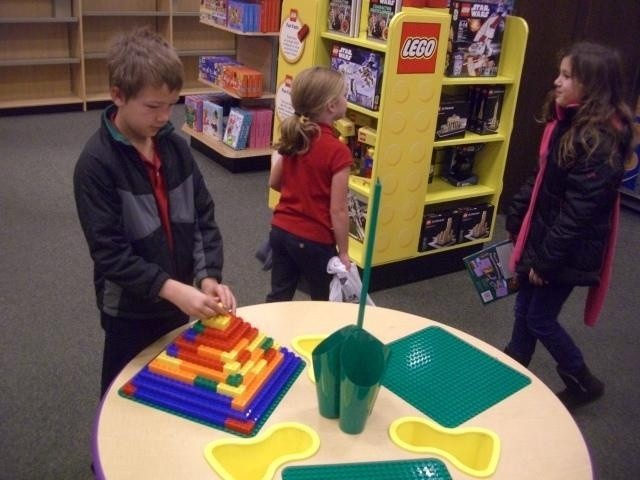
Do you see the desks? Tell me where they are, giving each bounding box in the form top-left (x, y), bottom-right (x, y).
top-left (90, 300), bottom-right (594, 479)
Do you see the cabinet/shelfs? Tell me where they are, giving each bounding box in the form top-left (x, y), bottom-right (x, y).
top-left (269, 0), bottom-right (531, 294)
top-left (180, 0), bottom-right (283, 174)
top-left (0, 0), bottom-right (237, 111)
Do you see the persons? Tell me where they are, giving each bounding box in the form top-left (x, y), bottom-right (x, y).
top-left (74, 23), bottom-right (237, 475)
top-left (263, 66), bottom-right (355, 303)
top-left (503, 41), bottom-right (635, 410)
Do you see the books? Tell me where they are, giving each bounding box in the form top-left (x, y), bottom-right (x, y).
top-left (463, 238), bottom-right (520, 306)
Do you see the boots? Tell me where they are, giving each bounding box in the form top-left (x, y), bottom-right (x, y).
top-left (555, 365), bottom-right (606, 410)
